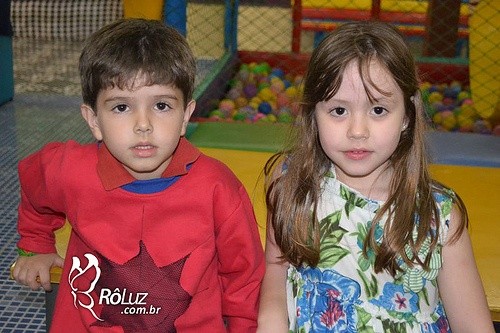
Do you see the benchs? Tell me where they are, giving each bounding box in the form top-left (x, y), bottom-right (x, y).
top-left (9, 145), bottom-right (500, 333)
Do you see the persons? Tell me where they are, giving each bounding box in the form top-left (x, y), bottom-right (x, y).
top-left (12, 17), bottom-right (265, 333)
top-left (254, 20), bottom-right (494, 331)
